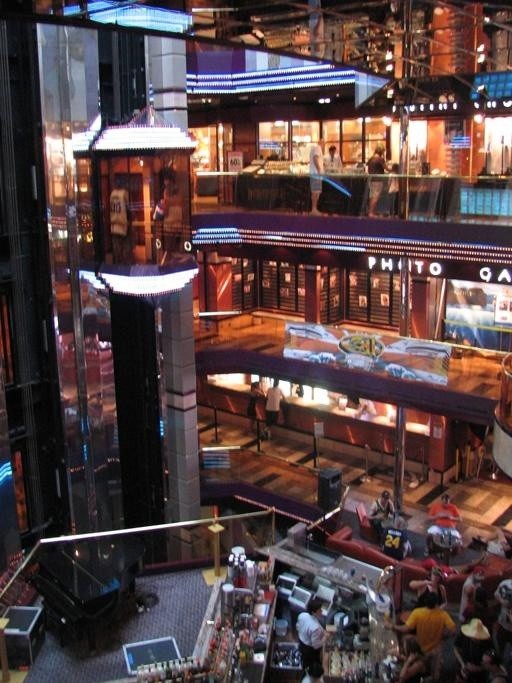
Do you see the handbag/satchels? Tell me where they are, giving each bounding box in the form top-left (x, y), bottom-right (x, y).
top-left (372, 500), bottom-right (385, 527)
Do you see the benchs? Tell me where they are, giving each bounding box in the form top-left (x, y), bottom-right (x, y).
top-left (355, 502), bottom-right (378, 541)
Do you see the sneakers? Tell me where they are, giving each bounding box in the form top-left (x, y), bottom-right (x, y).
top-left (437, 551), bottom-right (445, 564)
top-left (424, 544), bottom-right (432, 557)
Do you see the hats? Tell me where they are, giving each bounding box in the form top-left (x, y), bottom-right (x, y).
top-left (460, 618), bottom-right (491, 640)
top-left (428, 565), bottom-right (444, 583)
top-left (382, 490), bottom-right (391, 499)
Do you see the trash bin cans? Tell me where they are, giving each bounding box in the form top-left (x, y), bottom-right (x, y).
top-left (318, 468), bottom-right (342, 510)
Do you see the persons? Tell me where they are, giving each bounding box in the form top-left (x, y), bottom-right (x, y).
top-left (309, 137), bottom-right (328, 215)
top-left (245, 381), bottom-right (265, 432)
top-left (296, 601), bottom-right (330, 682)
top-left (324, 143), bottom-right (343, 166)
top-left (368, 489), bottom-right (397, 549)
top-left (364, 142), bottom-right (391, 218)
top-left (109, 178), bottom-right (135, 263)
top-left (386, 162), bottom-right (399, 215)
top-left (387, 557), bottom-right (511, 682)
top-left (354, 398), bottom-right (379, 421)
top-left (264, 378), bottom-right (286, 438)
top-left (153, 167), bottom-right (183, 265)
top-left (425, 494), bottom-right (461, 528)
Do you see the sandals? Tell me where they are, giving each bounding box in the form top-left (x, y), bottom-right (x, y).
top-left (471, 536), bottom-right (488, 546)
